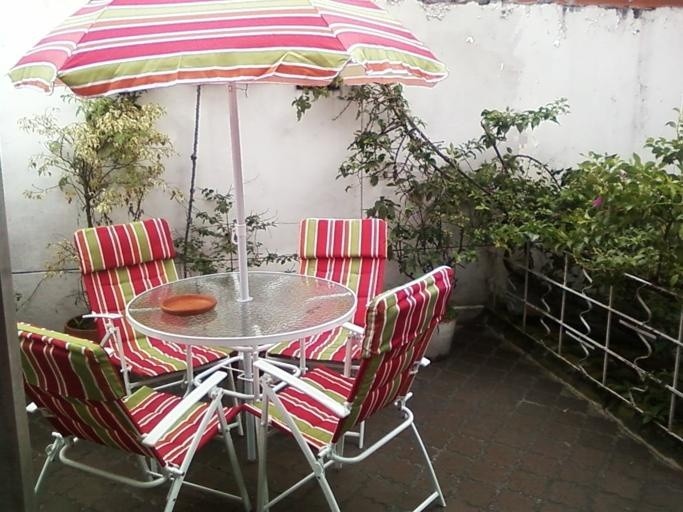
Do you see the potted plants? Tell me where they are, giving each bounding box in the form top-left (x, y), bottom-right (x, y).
top-left (15, 92), bottom-right (171, 342)
top-left (363, 169), bottom-right (460, 360)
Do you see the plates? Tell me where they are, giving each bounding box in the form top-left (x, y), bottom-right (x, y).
top-left (159, 293), bottom-right (218, 315)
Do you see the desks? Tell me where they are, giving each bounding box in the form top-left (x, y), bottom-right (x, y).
top-left (125, 270), bottom-right (357, 512)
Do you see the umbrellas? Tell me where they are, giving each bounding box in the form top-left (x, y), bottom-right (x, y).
top-left (7, 0), bottom-right (450, 460)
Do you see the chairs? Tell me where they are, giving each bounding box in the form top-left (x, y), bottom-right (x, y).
top-left (73, 218), bottom-right (243, 478)
top-left (245, 265), bottom-right (455, 512)
top-left (258, 216), bottom-right (388, 450)
top-left (16, 320), bottom-right (252, 512)
